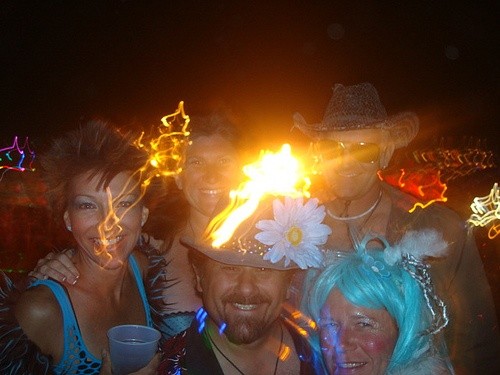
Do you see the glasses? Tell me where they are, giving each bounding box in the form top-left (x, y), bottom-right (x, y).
top-left (315, 139), bottom-right (380, 164)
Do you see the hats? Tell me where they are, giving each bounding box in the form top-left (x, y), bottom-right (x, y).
top-left (180, 190), bottom-right (298, 270)
top-left (293, 83), bottom-right (420, 150)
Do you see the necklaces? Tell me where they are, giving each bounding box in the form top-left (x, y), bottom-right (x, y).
top-left (326, 189), bottom-right (382, 221)
top-left (208, 324), bottom-right (284, 375)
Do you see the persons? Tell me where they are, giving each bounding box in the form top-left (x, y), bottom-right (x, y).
top-left (0, 73), bottom-right (499, 375)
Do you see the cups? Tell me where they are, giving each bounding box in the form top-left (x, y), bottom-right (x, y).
top-left (107, 325), bottom-right (162, 375)
